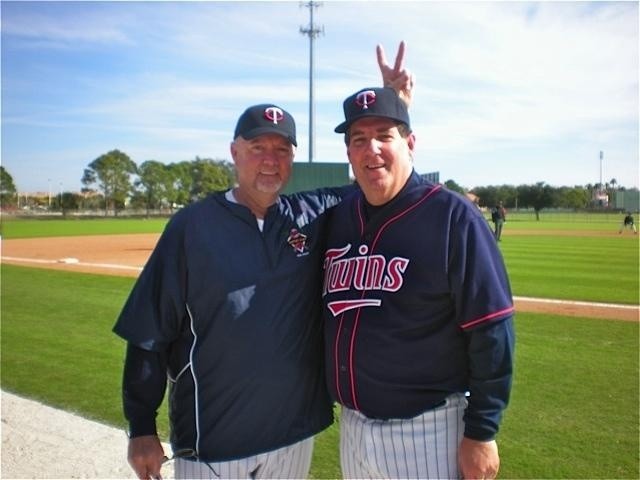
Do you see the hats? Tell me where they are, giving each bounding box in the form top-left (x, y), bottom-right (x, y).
top-left (233, 105), bottom-right (297, 147)
top-left (335, 88), bottom-right (410, 133)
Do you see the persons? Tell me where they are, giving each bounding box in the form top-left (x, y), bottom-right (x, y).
top-left (112, 40), bottom-right (415, 479)
top-left (323, 87), bottom-right (515, 479)
top-left (493, 200), bottom-right (506, 242)
top-left (619, 211), bottom-right (638, 235)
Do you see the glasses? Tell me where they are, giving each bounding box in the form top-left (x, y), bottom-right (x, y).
top-left (150, 449), bottom-right (221, 479)
